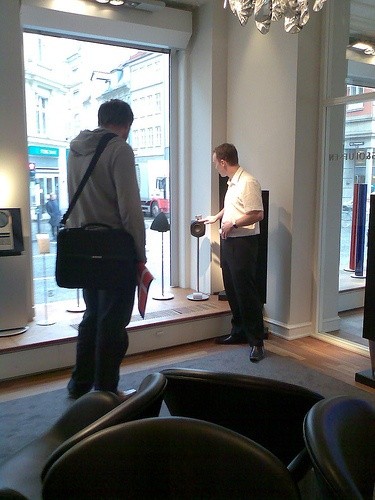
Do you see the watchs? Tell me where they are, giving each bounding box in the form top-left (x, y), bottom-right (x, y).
top-left (232, 218), bottom-right (238, 229)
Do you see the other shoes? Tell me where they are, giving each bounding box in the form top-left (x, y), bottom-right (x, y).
top-left (116, 387), bottom-right (137, 404)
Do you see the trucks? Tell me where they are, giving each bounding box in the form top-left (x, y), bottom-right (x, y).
top-left (135, 158), bottom-right (169, 219)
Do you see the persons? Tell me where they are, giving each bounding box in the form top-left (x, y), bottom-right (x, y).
top-left (197, 141), bottom-right (265, 362)
top-left (67, 98), bottom-right (156, 405)
top-left (46, 192), bottom-right (62, 241)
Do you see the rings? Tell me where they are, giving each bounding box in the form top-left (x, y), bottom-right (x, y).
top-left (223, 232), bottom-right (225, 234)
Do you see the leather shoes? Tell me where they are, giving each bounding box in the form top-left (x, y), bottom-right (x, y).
top-left (216, 334), bottom-right (249, 344)
top-left (250, 345), bottom-right (265, 362)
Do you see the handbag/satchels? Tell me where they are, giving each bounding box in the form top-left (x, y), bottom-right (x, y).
top-left (54, 226), bottom-right (87, 289)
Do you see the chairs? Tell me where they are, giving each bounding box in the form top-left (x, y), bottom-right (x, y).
top-left (0, 369), bottom-right (375, 500)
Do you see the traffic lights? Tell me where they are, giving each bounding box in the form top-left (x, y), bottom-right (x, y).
top-left (28, 163), bottom-right (36, 182)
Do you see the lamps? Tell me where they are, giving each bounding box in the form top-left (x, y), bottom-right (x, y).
top-left (224, 0), bottom-right (327, 35)
